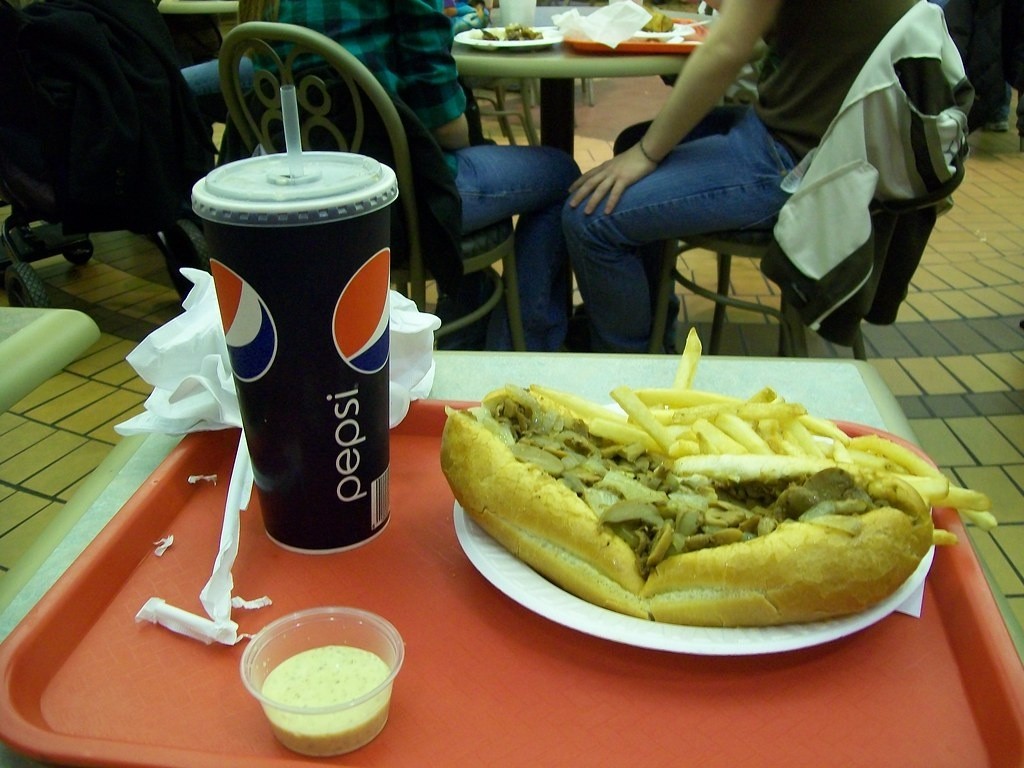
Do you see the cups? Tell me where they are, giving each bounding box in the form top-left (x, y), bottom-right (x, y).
top-left (191, 153), bottom-right (400, 553)
top-left (500, 0), bottom-right (537, 27)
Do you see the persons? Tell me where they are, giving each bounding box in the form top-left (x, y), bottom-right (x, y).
top-left (237, 0), bottom-right (582, 352)
top-left (0, 0), bottom-right (256, 235)
top-left (560, 0), bottom-right (922, 354)
top-left (933, 0), bottom-right (1013, 131)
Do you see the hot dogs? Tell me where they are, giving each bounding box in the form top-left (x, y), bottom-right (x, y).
top-left (440, 384), bottom-right (934, 628)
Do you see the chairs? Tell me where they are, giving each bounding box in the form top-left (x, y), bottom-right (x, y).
top-left (218, 21), bottom-right (528, 354)
top-left (646, 0), bottom-right (944, 360)
top-left (468, 74), bottom-right (541, 147)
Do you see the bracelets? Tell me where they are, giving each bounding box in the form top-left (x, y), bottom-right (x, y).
top-left (641, 137), bottom-right (659, 165)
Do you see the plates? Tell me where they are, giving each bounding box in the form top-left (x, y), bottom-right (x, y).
top-left (454, 26), bottom-right (565, 51)
top-left (635, 24), bottom-right (697, 40)
top-left (452, 499), bottom-right (936, 656)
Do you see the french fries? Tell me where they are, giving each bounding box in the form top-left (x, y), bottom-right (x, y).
top-left (608, 322), bottom-right (997, 546)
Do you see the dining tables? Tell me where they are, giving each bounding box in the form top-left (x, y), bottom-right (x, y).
top-left (450, 6), bottom-right (714, 159)
top-left (0, 350), bottom-right (1024, 768)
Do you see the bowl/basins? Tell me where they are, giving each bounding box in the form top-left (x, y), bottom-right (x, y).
top-left (240, 609), bottom-right (404, 755)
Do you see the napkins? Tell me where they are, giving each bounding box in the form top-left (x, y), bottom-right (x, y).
top-left (113, 267), bottom-right (444, 434)
top-left (549, 0), bottom-right (652, 49)
